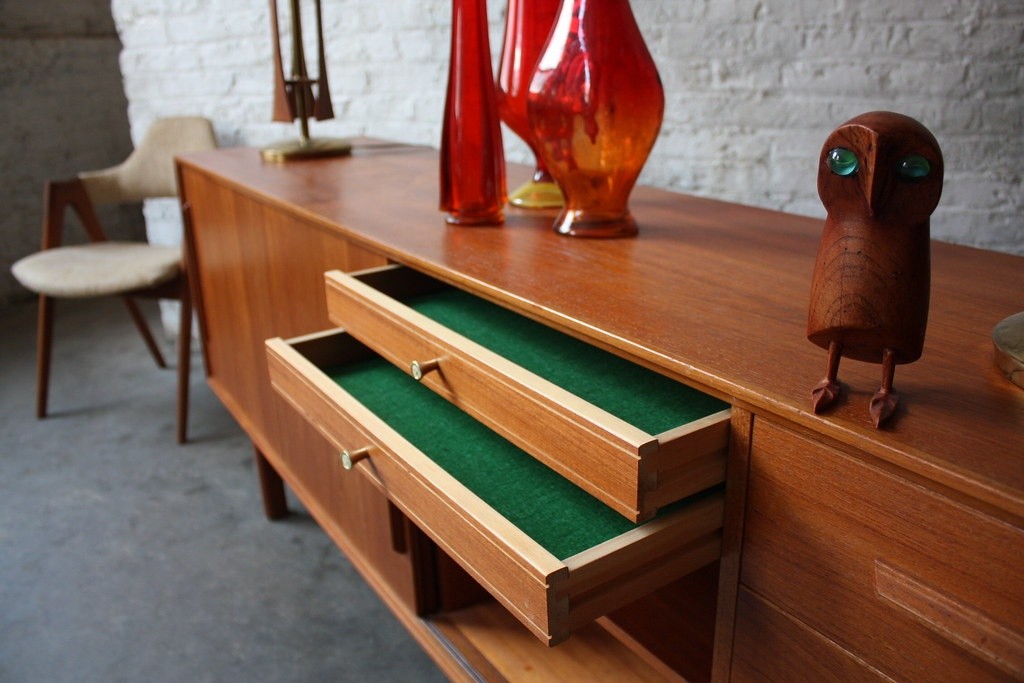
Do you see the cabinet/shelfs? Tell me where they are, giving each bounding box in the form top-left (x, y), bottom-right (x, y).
top-left (169, 136), bottom-right (1023, 683)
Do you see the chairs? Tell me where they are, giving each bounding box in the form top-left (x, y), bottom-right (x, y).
top-left (10, 117), bottom-right (229, 442)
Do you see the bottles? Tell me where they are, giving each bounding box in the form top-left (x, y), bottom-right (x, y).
top-left (441, 0), bottom-right (665, 239)
